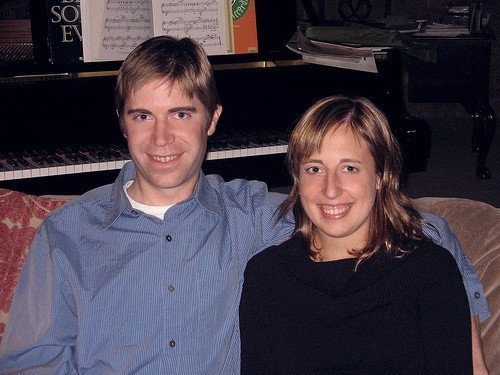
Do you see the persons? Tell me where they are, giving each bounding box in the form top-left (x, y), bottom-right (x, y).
top-left (239, 94), bottom-right (473, 375)
top-left (0, 35), bottom-right (493, 374)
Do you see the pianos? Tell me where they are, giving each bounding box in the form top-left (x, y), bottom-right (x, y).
top-left (1, 1), bottom-right (434, 197)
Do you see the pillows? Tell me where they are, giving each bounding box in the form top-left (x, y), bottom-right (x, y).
top-left (1, 188), bottom-right (67, 343)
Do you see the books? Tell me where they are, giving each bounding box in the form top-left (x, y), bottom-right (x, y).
top-left (81, 0), bottom-right (258, 62)
top-left (285, 5), bottom-right (469, 73)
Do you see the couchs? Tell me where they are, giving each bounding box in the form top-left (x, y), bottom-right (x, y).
top-left (0, 188), bottom-right (500, 375)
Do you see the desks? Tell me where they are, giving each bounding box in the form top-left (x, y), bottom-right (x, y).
top-left (304, 21), bottom-right (496, 180)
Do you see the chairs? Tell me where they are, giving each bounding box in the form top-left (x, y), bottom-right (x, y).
top-left (303, 0), bottom-right (391, 29)
